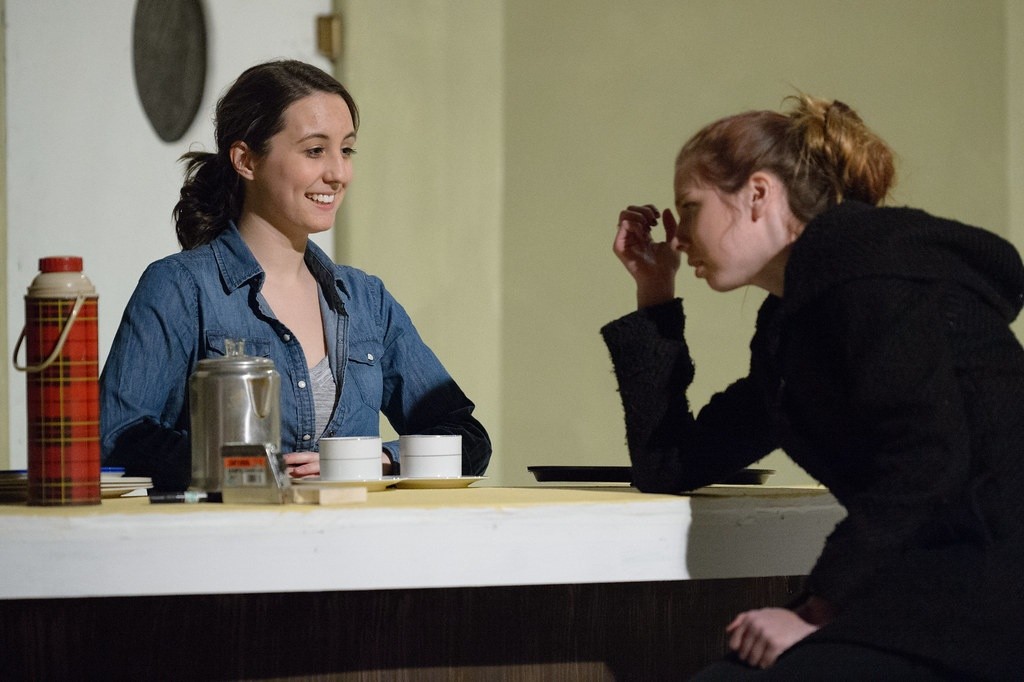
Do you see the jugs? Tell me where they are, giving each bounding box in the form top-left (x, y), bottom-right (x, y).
top-left (190, 338), bottom-right (281, 494)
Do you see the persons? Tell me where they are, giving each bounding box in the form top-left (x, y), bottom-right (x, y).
top-left (596, 82), bottom-right (1024, 682)
top-left (100, 59), bottom-right (494, 487)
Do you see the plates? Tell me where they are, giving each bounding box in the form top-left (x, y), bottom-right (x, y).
top-left (0, 467), bottom-right (154, 501)
top-left (395, 477), bottom-right (489, 489)
top-left (292, 477), bottom-right (403, 492)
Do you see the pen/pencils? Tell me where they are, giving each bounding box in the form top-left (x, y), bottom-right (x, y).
top-left (151, 494), bottom-right (208, 503)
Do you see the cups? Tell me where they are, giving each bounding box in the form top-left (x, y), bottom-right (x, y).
top-left (399, 435), bottom-right (462, 478)
top-left (319, 436), bottom-right (383, 481)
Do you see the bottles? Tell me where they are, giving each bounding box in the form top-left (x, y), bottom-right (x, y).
top-left (13, 257), bottom-right (102, 507)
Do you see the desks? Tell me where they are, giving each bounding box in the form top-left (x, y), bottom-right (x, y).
top-left (0, 480), bottom-right (850, 607)
